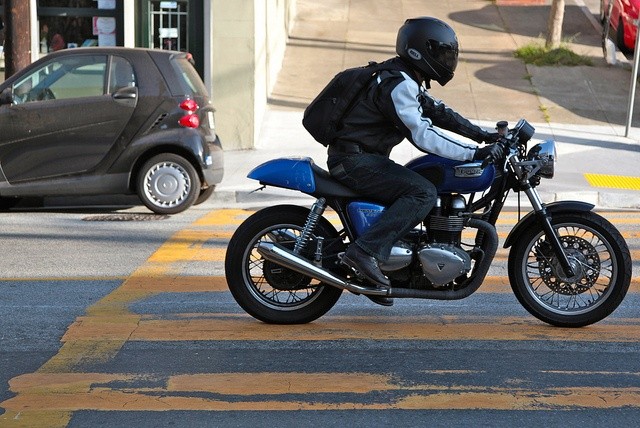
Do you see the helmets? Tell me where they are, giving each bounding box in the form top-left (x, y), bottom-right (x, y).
top-left (396, 16), bottom-right (458, 85)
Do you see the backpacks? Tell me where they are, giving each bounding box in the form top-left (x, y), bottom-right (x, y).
top-left (303, 61), bottom-right (418, 155)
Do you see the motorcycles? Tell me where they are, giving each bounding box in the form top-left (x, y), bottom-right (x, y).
top-left (224, 119), bottom-right (632, 328)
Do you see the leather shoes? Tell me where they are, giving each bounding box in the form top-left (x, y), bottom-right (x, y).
top-left (349, 289), bottom-right (393, 306)
top-left (342, 244), bottom-right (390, 287)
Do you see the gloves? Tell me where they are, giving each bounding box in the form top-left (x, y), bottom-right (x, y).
top-left (483, 132), bottom-right (499, 143)
top-left (475, 144), bottom-right (503, 163)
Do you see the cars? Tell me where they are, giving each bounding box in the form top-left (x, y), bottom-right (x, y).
top-left (601, 0), bottom-right (640, 53)
top-left (0, 47), bottom-right (224, 215)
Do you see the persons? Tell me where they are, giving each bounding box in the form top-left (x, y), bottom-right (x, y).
top-left (328, 16), bottom-right (505, 307)
